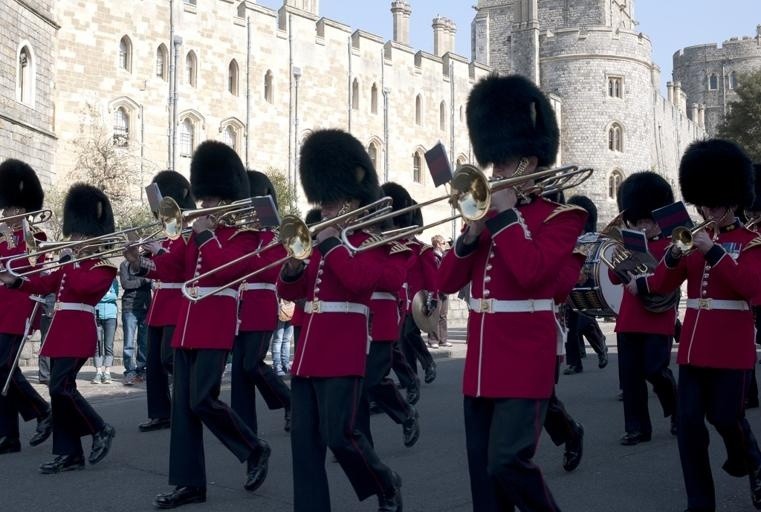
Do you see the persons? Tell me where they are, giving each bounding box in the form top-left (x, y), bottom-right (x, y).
top-left (608, 202), bottom-right (761, 512)
top-left (1, 154), bottom-right (447, 510)
top-left (431, 154), bottom-right (609, 511)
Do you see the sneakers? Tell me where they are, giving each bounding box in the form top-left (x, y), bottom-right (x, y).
top-left (273, 364), bottom-right (295, 377)
top-left (90, 372), bottom-right (113, 385)
top-left (429, 341), bottom-right (453, 348)
top-left (123, 374), bottom-right (140, 385)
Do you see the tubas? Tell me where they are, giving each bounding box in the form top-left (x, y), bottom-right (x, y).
top-left (598, 212), bottom-right (681, 313)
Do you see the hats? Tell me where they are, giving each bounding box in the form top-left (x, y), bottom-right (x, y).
top-left (678, 138), bottom-right (756, 209)
top-left (63, 184), bottom-right (115, 237)
top-left (466, 73), bottom-right (559, 169)
top-left (190, 141), bottom-right (249, 200)
top-left (152, 171), bottom-right (196, 211)
top-left (621, 171), bottom-right (674, 215)
top-left (411, 198), bottom-right (423, 234)
top-left (0, 158), bottom-right (44, 211)
top-left (304, 208), bottom-right (324, 240)
top-left (751, 161), bottom-right (760, 211)
top-left (247, 170), bottom-right (278, 213)
top-left (567, 195), bottom-right (597, 232)
top-left (617, 180), bottom-right (626, 213)
top-left (299, 129), bottom-right (378, 200)
top-left (380, 182), bottom-right (413, 226)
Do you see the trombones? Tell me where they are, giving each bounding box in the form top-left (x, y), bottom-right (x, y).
top-left (341, 166), bottom-right (593, 252)
top-left (183, 196), bottom-right (392, 303)
top-left (1, 197), bottom-right (259, 276)
top-left (0, 208), bottom-right (50, 242)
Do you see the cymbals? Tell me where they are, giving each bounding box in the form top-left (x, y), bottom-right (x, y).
top-left (413, 290), bottom-right (442, 333)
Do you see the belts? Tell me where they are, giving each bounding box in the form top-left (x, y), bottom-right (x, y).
top-left (53, 301), bottom-right (95, 314)
top-left (370, 292), bottom-right (397, 301)
top-left (201, 287), bottom-right (237, 299)
top-left (686, 298), bottom-right (750, 313)
top-left (303, 300), bottom-right (367, 315)
top-left (241, 283), bottom-right (276, 292)
top-left (152, 281), bottom-right (185, 290)
top-left (469, 296), bottom-right (553, 314)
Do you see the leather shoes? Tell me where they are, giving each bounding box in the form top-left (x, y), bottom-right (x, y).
top-left (424, 359), bottom-right (436, 384)
top-left (748, 466), bottom-right (761, 509)
top-left (0, 435), bottom-right (22, 455)
top-left (379, 469), bottom-right (403, 512)
top-left (152, 480), bottom-right (209, 509)
top-left (283, 405), bottom-right (296, 432)
top-left (562, 360), bottom-right (584, 374)
top-left (402, 404), bottom-right (419, 448)
top-left (38, 447), bottom-right (85, 474)
top-left (88, 423), bottom-right (116, 464)
top-left (612, 384), bottom-right (760, 446)
top-left (597, 341), bottom-right (609, 369)
top-left (139, 415), bottom-right (170, 433)
top-left (29, 421), bottom-right (52, 447)
top-left (407, 375), bottom-right (422, 405)
top-left (562, 420), bottom-right (584, 471)
top-left (244, 442), bottom-right (273, 493)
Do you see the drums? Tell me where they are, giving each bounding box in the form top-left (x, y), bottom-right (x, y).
top-left (568, 234), bottom-right (625, 317)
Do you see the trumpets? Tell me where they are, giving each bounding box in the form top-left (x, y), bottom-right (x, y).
top-left (665, 219), bottom-right (719, 258)
